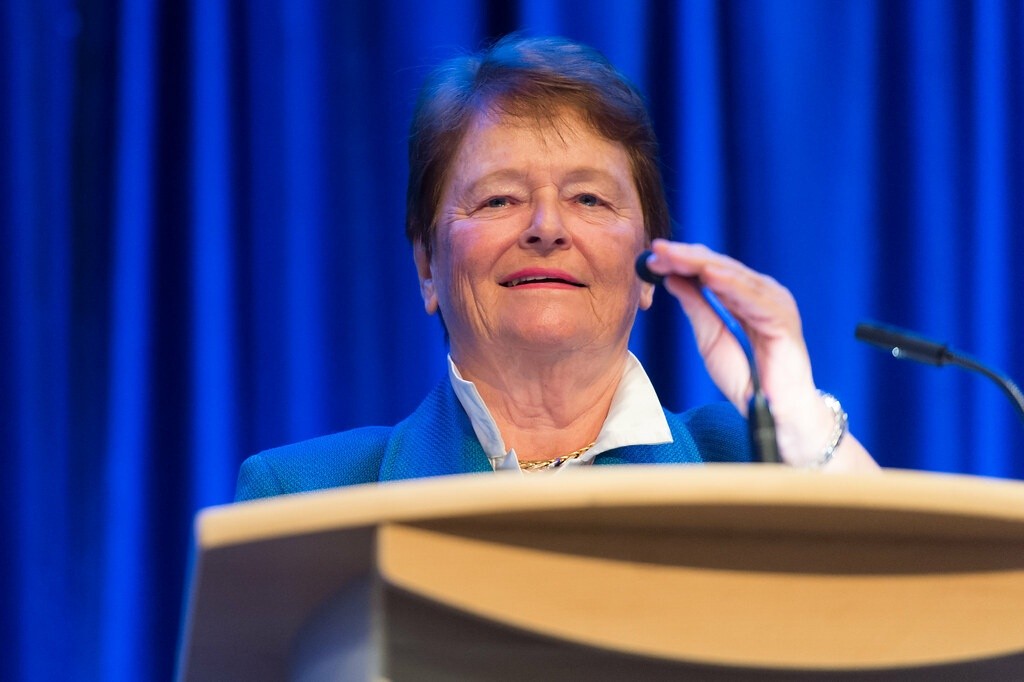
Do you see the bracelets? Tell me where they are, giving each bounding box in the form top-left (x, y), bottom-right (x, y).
top-left (810, 388), bottom-right (848, 467)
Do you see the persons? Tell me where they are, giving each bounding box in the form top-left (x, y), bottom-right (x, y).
top-left (233, 36), bottom-right (883, 506)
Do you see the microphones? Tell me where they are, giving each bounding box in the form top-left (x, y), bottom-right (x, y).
top-left (634, 249), bottom-right (779, 463)
top-left (855, 322), bottom-right (1024, 418)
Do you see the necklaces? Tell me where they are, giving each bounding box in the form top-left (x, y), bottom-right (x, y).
top-left (519, 441), bottom-right (596, 472)
